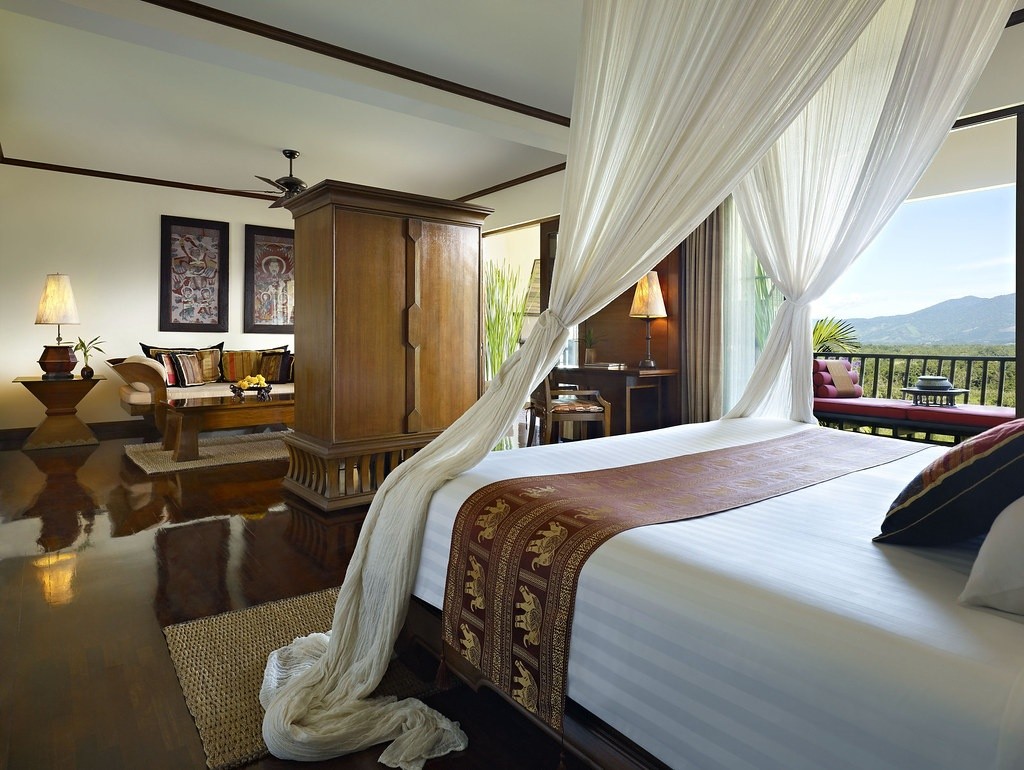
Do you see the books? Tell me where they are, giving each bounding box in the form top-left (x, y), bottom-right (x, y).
top-left (584, 362), bottom-right (625, 366)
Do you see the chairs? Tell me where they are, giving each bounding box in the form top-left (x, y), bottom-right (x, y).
top-left (526, 372), bottom-right (611, 446)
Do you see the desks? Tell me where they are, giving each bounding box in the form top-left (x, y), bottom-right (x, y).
top-left (548, 368), bottom-right (677, 433)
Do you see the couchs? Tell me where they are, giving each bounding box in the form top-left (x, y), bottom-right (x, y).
top-left (103, 351), bottom-right (296, 443)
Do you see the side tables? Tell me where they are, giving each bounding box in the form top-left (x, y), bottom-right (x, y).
top-left (12, 374), bottom-right (103, 450)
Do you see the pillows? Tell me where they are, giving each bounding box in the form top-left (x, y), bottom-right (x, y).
top-left (955, 499), bottom-right (1024, 613)
top-left (870, 418), bottom-right (1024, 548)
top-left (139, 342), bottom-right (294, 387)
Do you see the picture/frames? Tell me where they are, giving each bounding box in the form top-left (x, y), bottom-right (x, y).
top-left (242, 224), bottom-right (295, 334)
top-left (158, 215), bottom-right (229, 332)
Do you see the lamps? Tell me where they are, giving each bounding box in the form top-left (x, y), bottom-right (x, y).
top-left (34, 272), bottom-right (80, 379)
top-left (630, 271), bottom-right (667, 369)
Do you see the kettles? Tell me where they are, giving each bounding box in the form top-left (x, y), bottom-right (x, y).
top-left (564, 340), bottom-right (578, 365)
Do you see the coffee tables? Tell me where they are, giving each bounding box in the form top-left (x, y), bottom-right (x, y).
top-left (155, 393), bottom-right (296, 462)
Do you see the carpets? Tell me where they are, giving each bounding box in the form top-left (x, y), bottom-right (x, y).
top-left (123, 429), bottom-right (294, 473)
top-left (161, 582), bottom-right (403, 770)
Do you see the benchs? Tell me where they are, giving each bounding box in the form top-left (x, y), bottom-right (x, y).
top-left (814, 358), bottom-right (1017, 444)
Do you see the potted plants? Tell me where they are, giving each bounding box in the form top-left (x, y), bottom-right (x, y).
top-left (75, 335), bottom-right (106, 379)
top-left (576, 328), bottom-right (607, 363)
top-left (480, 261), bottom-right (533, 452)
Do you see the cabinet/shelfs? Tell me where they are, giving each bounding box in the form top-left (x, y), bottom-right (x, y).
top-left (285, 179), bottom-right (495, 510)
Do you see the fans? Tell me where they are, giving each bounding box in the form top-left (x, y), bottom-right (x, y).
top-left (235, 149), bottom-right (307, 209)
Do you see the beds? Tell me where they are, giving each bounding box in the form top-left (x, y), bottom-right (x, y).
top-left (383, 413), bottom-right (1023, 769)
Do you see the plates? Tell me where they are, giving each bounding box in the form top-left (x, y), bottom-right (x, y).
top-left (230, 383), bottom-right (273, 403)
